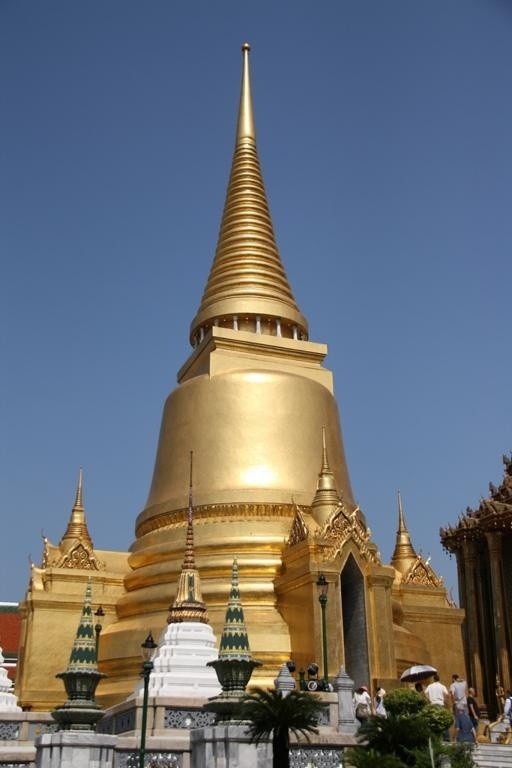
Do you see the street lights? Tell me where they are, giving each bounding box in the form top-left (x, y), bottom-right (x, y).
top-left (94, 605), bottom-right (106, 660)
top-left (139, 630), bottom-right (157, 768)
top-left (318, 569), bottom-right (330, 681)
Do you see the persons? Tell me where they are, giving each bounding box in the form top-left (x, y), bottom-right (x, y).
top-left (287, 660), bottom-right (511, 751)
